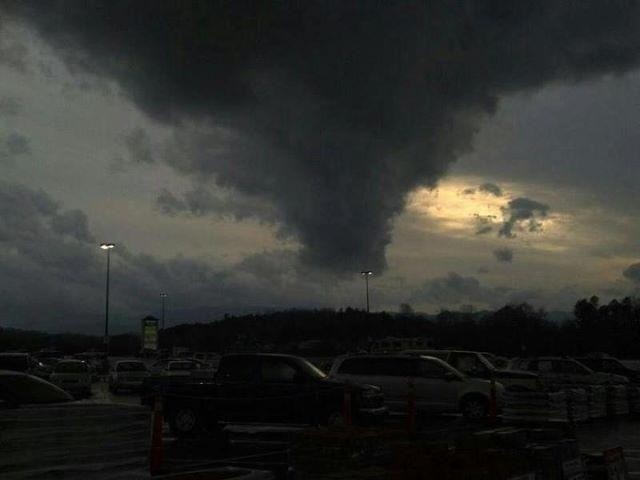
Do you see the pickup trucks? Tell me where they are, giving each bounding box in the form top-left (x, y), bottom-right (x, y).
top-left (106, 360), bottom-right (147, 393)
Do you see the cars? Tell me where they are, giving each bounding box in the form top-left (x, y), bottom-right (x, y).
top-left (0, 348), bottom-right (110, 413)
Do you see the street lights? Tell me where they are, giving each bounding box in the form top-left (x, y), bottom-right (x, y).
top-left (99, 241), bottom-right (118, 375)
top-left (159, 289), bottom-right (168, 329)
top-left (361, 270), bottom-right (373, 313)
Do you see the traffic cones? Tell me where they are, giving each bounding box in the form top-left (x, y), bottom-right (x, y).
top-left (148, 414), bottom-right (169, 474)
